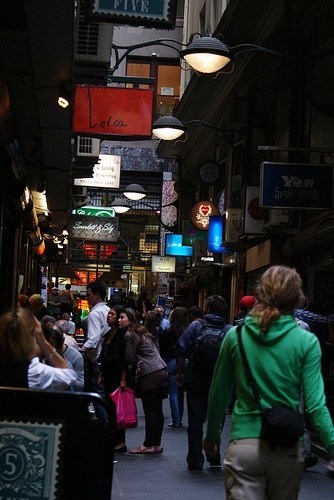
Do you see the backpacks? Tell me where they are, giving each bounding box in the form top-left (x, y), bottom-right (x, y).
top-left (184, 321), bottom-right (226, 394)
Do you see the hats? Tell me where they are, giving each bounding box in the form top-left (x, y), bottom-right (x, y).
top-left (240, 296), bottom-right (255, 309)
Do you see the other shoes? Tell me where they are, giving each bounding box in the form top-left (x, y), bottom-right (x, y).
top-left (169, 420), bottom-right (183, 427)
top-left (129, 445), bottom-right (164, 453)
top-left (114, 445), bottom-right (127, 453)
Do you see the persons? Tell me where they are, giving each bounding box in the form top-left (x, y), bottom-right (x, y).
top-left (0, 281), bottom-right (334, 471)
top-left (202, 265), bottom-right (334, 500)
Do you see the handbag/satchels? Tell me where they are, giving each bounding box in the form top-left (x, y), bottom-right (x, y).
top-left (109, 386), bottom-right (138, 429)
top-left (258, 403), bottom-right (305, 442)
